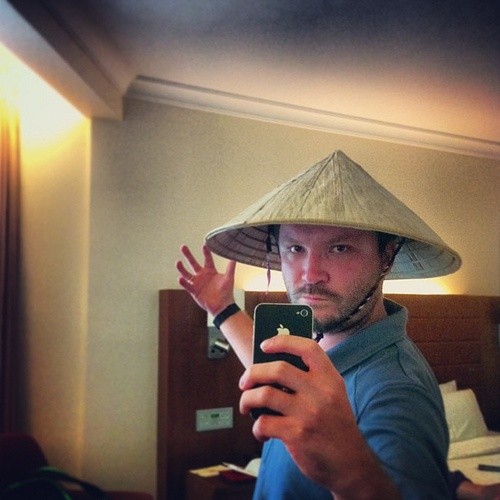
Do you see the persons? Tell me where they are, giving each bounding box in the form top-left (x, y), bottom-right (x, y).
top-left (176, 149), bottom-right (461, 500)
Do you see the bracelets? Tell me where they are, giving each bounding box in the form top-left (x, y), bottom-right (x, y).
top-left (213, 302), bottom-right (240, 330)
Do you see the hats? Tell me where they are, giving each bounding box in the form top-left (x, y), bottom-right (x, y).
top-left (203, 148), bottom-right (463, 280)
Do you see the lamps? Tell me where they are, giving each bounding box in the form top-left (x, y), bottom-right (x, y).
top-left (207, 288), bottom-right (246, 357)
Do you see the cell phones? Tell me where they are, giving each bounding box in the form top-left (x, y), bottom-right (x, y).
top-left (249, 302), bottom-right (314, 420)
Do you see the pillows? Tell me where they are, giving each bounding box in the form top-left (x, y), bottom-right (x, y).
top-left (440, 381), bottom-right (490, 443)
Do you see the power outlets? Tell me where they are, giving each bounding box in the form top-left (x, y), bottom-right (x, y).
top-left (195, 407), bottom-right (234, 433)
